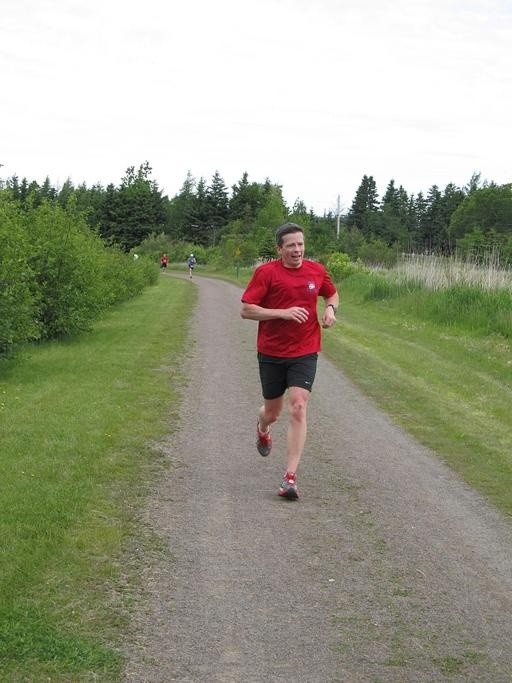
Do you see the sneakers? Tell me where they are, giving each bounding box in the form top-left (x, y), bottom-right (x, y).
top-left (256, 415), bottom-right (272, 456)
top-left (277, 471), bottom-right (301, 499)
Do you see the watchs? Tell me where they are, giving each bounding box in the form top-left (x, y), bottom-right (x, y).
top-left (327, 302), bottom-right (338, 314)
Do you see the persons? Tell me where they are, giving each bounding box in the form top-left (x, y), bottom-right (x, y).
top-left (159, 253), bottom-right (169, 272)
top-left (237, 220), bottom-right (340, 501)
top-left (186, 253), bottom-right (197, 278)
